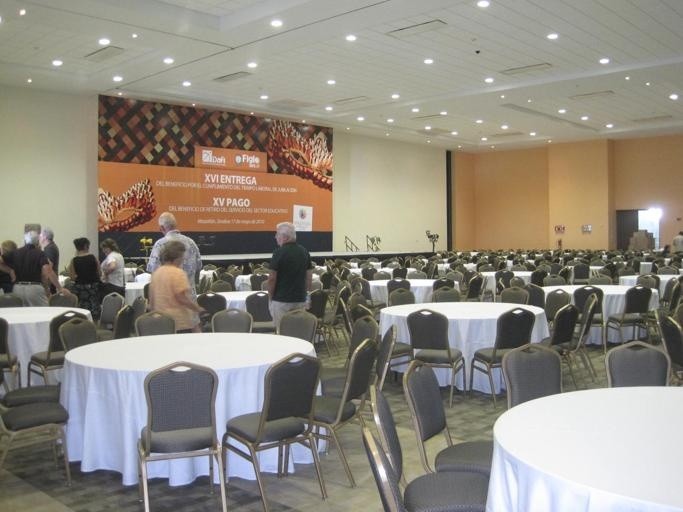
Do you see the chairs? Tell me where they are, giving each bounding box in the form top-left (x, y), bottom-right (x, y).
top-left (222, 353), bottom-right (329, 512)
top-left (0, 248), bottom-right (683, 486)
top-left (136, 360), bottom-right (226, 512)
top-left (363, 426), bottom-right (489, 511)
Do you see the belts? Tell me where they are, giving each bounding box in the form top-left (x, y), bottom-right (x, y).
top-left (14, 282), bottom-right (41, 286)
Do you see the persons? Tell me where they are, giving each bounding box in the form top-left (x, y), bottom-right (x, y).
top-left (144, 211), bottom-right (203, 305)
top-left (38, 228), bottom-right (59, 296)
top-left (661, 245), bottom-right (671, 257)
top-left (66, 236), bottom-right (107, 320)
top-left (145, 240), bottom-right (206, 335)
top-left (266, 222), bottom-right (315, 332)
top-left (671, 230), bottom-right (683, 255)
top-left (0, 228), bottom-right (73, 308)
top-left (93, 236), bottom-right (125, 305)
top-left (0, 240), bottom-right (18, 296)
